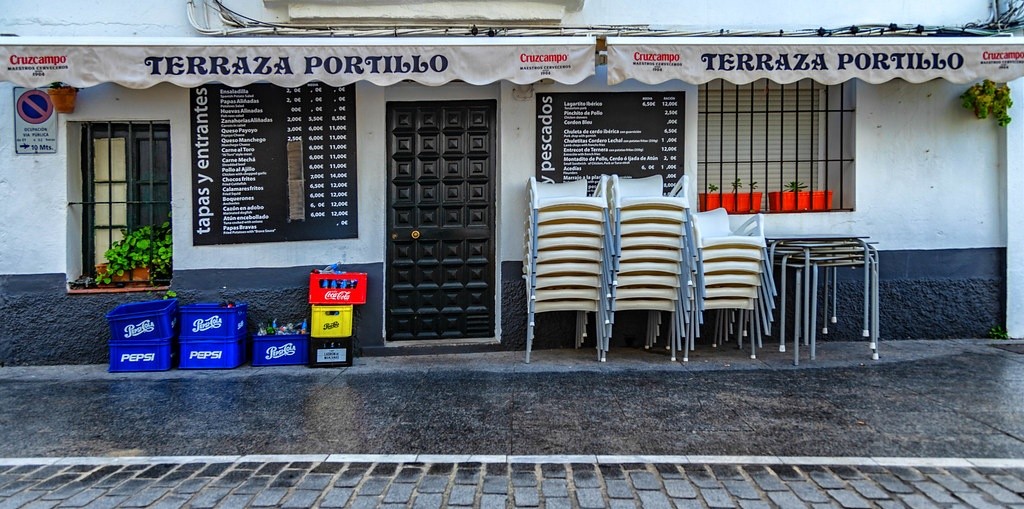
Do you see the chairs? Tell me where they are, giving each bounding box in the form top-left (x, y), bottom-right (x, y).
top-left (520, 174), bottom-right (779, 365)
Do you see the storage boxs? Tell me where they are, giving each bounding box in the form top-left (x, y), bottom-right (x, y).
top-left (308, 327), bottom-right (356, 366)
top-left (107, 298), bottom-right (179, 339)
top-left (311, 303), bottom-right (353, 338)
top-left (108, 340), bottom-right (172, 373)
top-left (250, 331), bottom-right (309, 367)
top-left (178, 336), bottom-right (246, 369)
top-left (181, 303), bottom-right (248, 339)
top-left (308, 271), bottom-right (367, 304)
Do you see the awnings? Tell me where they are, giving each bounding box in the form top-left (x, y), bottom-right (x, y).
top-left (0, 36), bottom-right (597, 89)
top-left (606, 33), bottom-right (1024, 86)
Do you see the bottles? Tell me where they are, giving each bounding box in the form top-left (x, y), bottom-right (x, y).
top-left (320, 261), bottom-right (342, 272)
top-left (320, 342), bottom-right (342, 349)
top-left (258, 319), bottom-right (307, 335)
top-left (319, 271), bottom-right (358, 289)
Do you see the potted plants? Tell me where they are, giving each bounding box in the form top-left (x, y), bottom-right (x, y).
top-left (699, 179), bottom-right (762, 213)
top-left (961, 79), bottom-right (1014, 128)
top-left (49, 81), bottom-right (77, 112)
top-left (767, 181), bottom-right (833, 212)
top-left (93, 221), bottom-right (172, 285)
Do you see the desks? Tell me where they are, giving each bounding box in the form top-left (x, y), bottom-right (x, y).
top-left (762, 234), bottom-right (881, 365)
